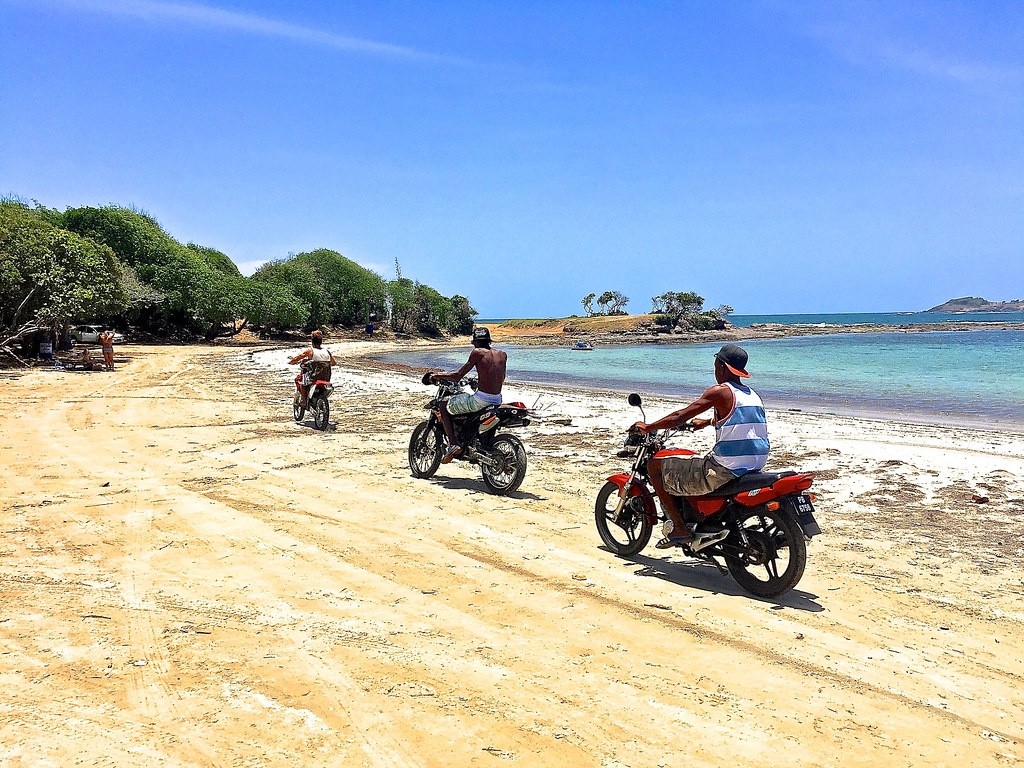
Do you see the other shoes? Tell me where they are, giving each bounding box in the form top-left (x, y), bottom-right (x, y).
top-left (441, 445), bottom-right (462, 464)
top-left (655, 533), bottom-right (690, 549)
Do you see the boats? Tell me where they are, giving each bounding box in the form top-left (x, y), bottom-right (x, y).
top-left (572, 342), bottom-right (594, 350)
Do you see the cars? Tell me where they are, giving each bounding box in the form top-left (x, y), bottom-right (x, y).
top-left (68, 324), bottom-right (125, 344)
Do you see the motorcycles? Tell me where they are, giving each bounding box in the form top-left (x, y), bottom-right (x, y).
top-left (287, 355), bottom-right (334, 430)
top-left (408, 373), bottom-right (532, 497)
top-left (594, 392), bottom-right (823, 599)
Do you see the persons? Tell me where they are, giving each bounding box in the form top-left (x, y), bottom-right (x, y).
top-left (82, 331), bottom-right (115, 372)
top-left (576, 339), bottom-right (584, 348)
top-left (289, 330), bottom-right (336, 407)
top-left (429, 327), bottom-right (508, 464)
top-left (629, 345), bottom-right (770, 549)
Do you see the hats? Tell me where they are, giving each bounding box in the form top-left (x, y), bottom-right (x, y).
top-left (473, 327), bottom-right (491, 341)
top-left (713, 343), bottom-right (751, 379)
